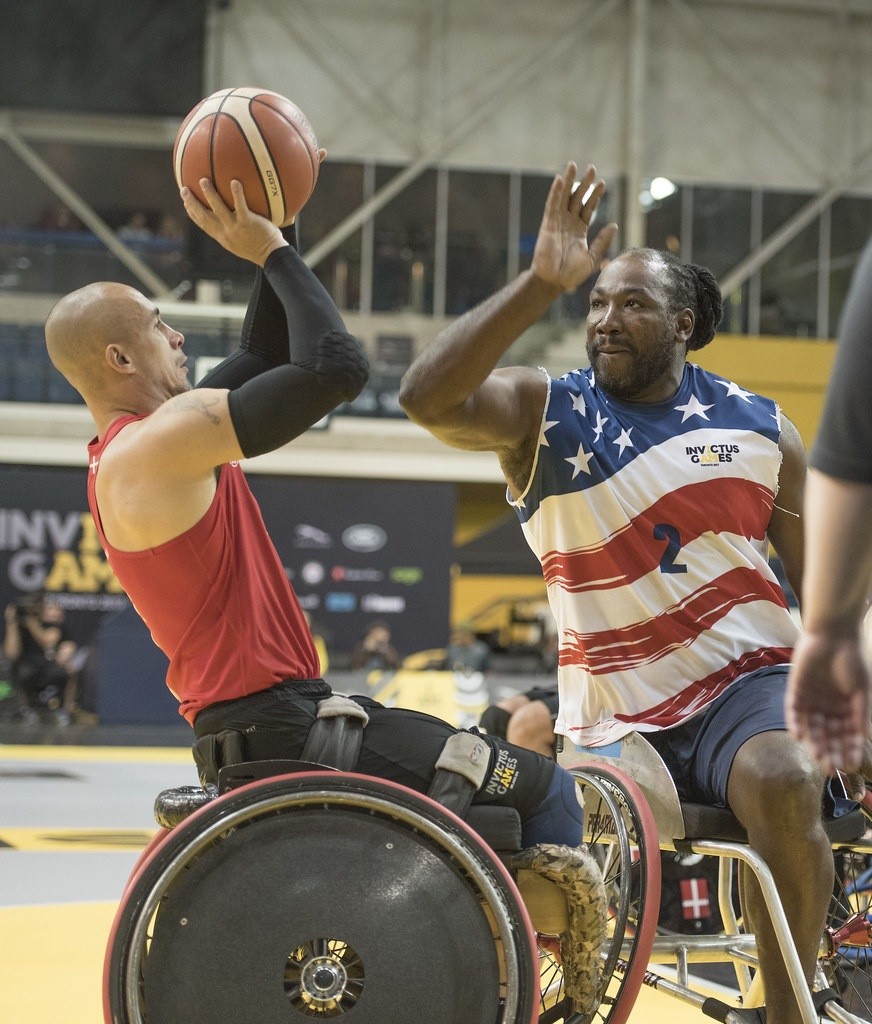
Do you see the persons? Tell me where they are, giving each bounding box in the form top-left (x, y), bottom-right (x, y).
top-left (40, 199), bottom-right (77, 231)
top-left (478, 684), bottom-right (559, 759)
top-left (302, 608), bottom-right (329, 677)
top-left (115, 211), bottom-right (153, 238)
top-left (784, 239), bottom-right (872, 777)
top-left (350, 621), bottom-right (400, 672)
top-left (45, 148), bottom-right (585, 935)
top-left (399, 161), bottom-right (872, 1024)
top-left (5, 588), bottom-right (84, 727)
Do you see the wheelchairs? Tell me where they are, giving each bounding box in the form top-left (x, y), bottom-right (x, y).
top-left (538, 731), bottom-right (872, 1023)
top-left (103, 727), bottom-right (573, 1024)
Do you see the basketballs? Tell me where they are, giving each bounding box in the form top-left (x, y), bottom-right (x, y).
top-left (174, 87), bottom-right (319, 227)
